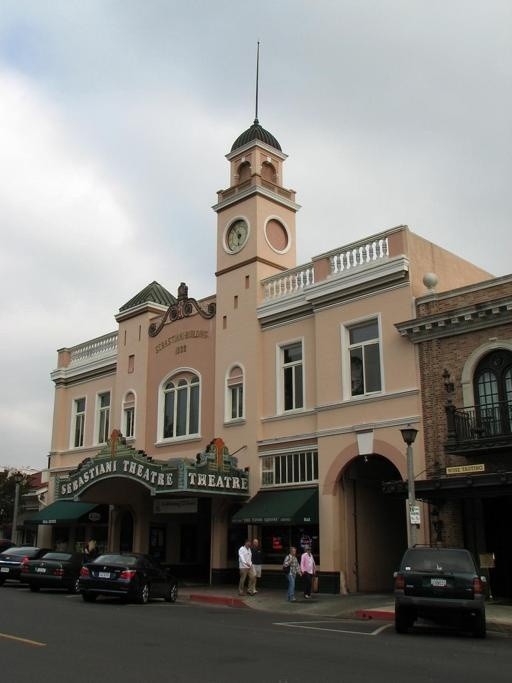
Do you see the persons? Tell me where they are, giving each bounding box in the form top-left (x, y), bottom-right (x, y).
top-left (250, 538), bottom-right (263, 591)
top-left (238, 540), bottom-right (256, 595)
top-left (300, 545), bottom-right (316, 599)
top-left (283, 547), bottom-right (302, 602)
top-left (84, 538), bottom-right (100, 563)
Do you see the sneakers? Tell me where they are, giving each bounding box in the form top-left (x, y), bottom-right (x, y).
top-left (304, 594), bottom-right (312, 599)
top-left (239, 589), bottom-right (258, 596)
top-left (288, 597), bottom-right (296, 601)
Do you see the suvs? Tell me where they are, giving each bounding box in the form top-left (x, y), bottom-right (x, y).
top-left (393, 542), bottom-right (490, 640)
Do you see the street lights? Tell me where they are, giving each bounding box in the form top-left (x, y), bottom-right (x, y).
top-left (399, 422), bottom-right (421, 547)
top-left (10, 470), bottom-right (24, 543)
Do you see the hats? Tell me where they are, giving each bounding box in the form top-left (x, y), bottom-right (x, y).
top-left (303, 545), bottom-right (311, 551)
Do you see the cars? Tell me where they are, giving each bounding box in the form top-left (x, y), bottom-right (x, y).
top-left (79, 551), bottom-right (177, 605)
top-left (20, 552), bottom-right (89, 596)
top-left (0, 539), bottom-right (16, 552)
top-left (0, 546), bottom-right (52, 587)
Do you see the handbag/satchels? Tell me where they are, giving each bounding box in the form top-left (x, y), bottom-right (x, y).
top-left (311, 576), bottom-right (319, 592)
top-left (282, 565), bottom-right (290, 574)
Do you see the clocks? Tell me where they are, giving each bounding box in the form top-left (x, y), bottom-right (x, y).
top-left (222, 216), bottom-right (249, 255)
